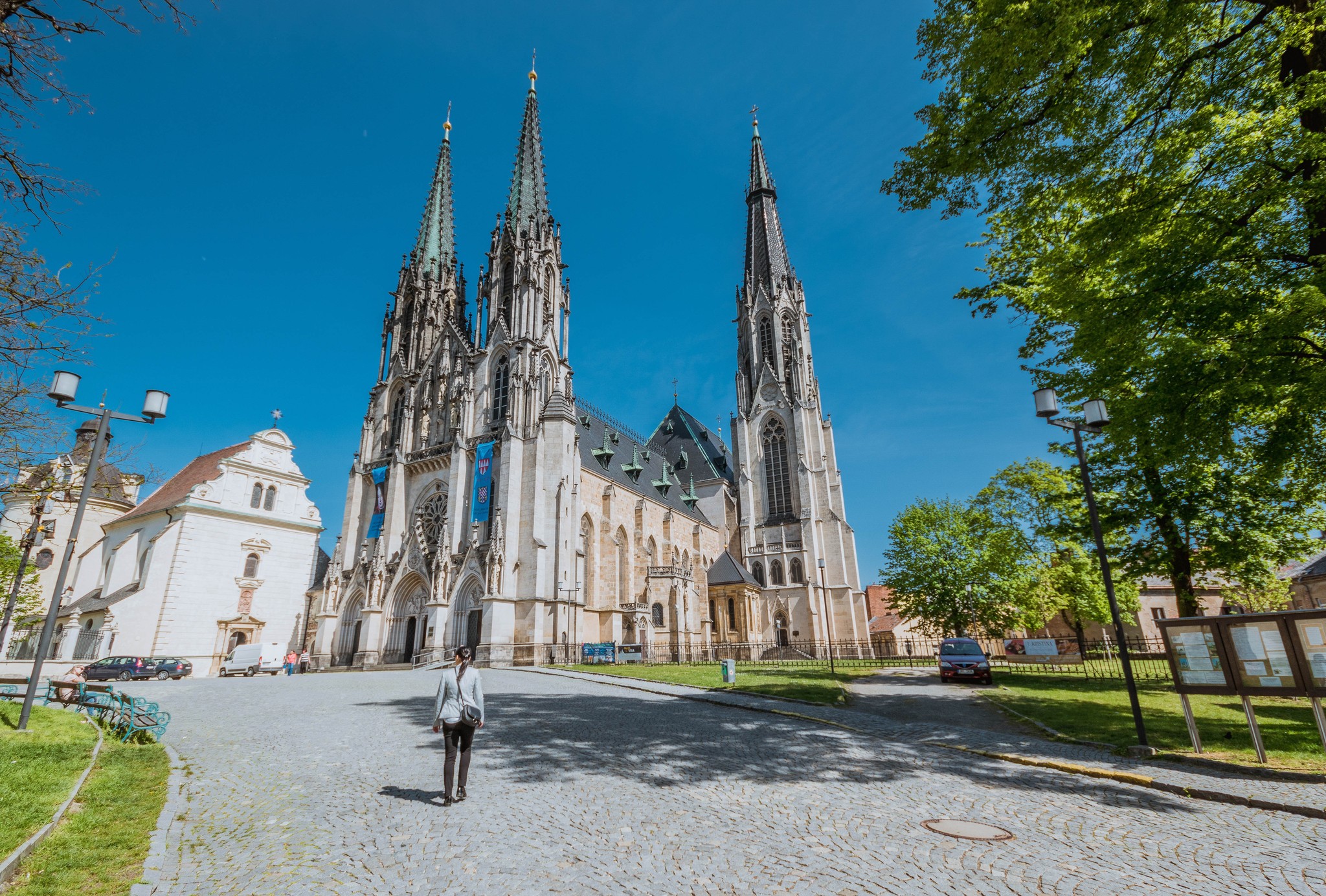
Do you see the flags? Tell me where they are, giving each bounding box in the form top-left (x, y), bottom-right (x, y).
top-left (366, 465), bottom-right (388, 539)
top-left (471, 440), bottom-right (496, 523)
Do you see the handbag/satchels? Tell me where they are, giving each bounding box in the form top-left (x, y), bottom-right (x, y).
top-left (307, 664), bottom-right (311, 672)
top-left (460, 704), bottom-right (482, 728)
top-left (69, 689), bottom-right (85, 703)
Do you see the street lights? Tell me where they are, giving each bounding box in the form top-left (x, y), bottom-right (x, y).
top-left (15, 369), bottom-right (170, 732)
top-left (965, 584), bottom-right (979, 644)
top-left (775, 620), bottom-right (783, 663)
top-left (674, 604), bottom-right (681, 666)
top-left (558, 582), bottom-right (581, 667)
top-left (1031, 386), bottom-right (1149, 747)
top-left (817, 558), bottom-right (835, 673)
top-left (918, 605), bottom-right (931, 668)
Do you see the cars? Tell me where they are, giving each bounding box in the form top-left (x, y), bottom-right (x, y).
top-left (131, 657), bottom-right (192, 681)
top-left (937, 637), bottom-right (993, 685)
top-left (78, 655), bottom-right (157, 682)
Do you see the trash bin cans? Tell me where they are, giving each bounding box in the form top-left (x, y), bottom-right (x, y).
top-left (720, 659), bottom-right (735, 683)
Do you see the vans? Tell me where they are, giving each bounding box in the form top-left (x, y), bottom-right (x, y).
top-left (218, 642), bottom-right (285, 678)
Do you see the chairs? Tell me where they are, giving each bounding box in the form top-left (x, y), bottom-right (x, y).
top-left (128, 659), bottom-right (132, 663)
top-left (44, 677), bottom-right (88, 716)
top-left (158, 661), bottom-right (162, 665)
top-left (116, 659), bottom-right (121, 664)
top-left (113, 690), bottom-right (172, 745)
top-left (945, 646), bottom-right (954, 654)
top-left (105, 688), bottom-right (159, 737)
top-left (0, 676), bottom-right (54, 705)
top-left (78, 679), bottom-right (126, 728)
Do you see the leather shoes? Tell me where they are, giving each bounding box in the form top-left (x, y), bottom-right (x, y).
top-left (456, 786), bottom-right (467, 797)
top-left (443, 796), bottom-right (452, 807)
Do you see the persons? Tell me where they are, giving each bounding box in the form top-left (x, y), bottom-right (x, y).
top-left (59, 665), bottom-right (98, 718)
top-left (326, 582), bottom-right (338, 607)
top-left (450, 403), bottom-right (459, 427)
top-left (421, 410), bottom-right (430, 437)
top-left (437, 379), bottom-right (448, 403)
top-left (298, 649), bottom-right (311, 676)
top-left (286, 650), bottom-right (297, 676)
top-left (373, 482), bottom-right (386, 515)
top-left (371, 577), bottom-right (381, 600)
top-left (489, 564), bottom-right (500, 587)
top-left (436, 571), bottom-right (445, 592)
top-left (432, 647), bottom-right (484, 806)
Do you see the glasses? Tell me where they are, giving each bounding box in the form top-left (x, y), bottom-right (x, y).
top-left (77, 667), bottom-right (83, 670)
